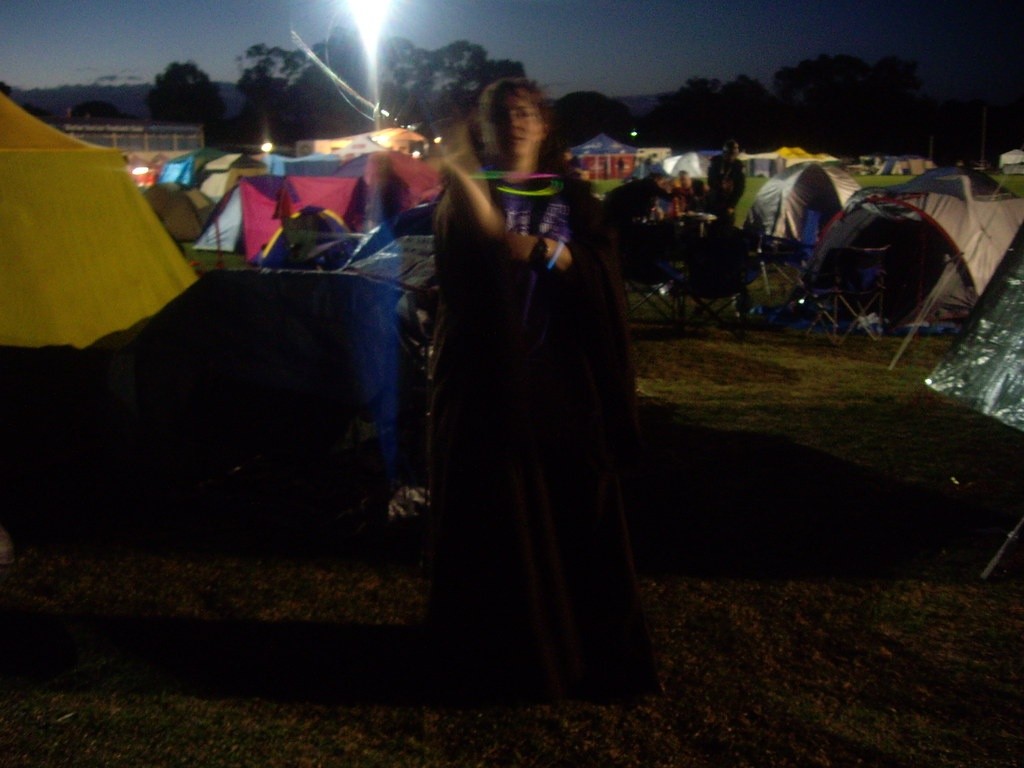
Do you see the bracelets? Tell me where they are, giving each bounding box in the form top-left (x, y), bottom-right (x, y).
top-left (546, 242), bottom-right (562, 268)
top-left (531, 238), bottom-right (547, 268)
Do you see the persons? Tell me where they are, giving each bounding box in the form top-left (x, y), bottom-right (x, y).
top-left (617, 157), bottom-right (624, 176)
top-left (568, 156), bottom-right (590, 180)
top-left (603, 139), bottom-right (744, 287)
top-left (377, 80), bottom-right (671, 719)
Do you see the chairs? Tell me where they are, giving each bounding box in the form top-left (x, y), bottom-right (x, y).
top-left (614, 237), bottom-right (891, 346)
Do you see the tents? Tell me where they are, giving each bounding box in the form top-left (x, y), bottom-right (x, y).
top-left (744, 164), bottom-right (869, 263)
top-left (780, 196), bottom-right (979, 335)
top-left (567, 133), bottom-right (637, 175)
top-left (898, 168), bottom-right (1023, 294)
top-left (0, 94), bottom-right (435, 535)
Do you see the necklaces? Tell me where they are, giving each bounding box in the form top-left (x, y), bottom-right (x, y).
top-left (495, 181), bottom-right (562, 196)
